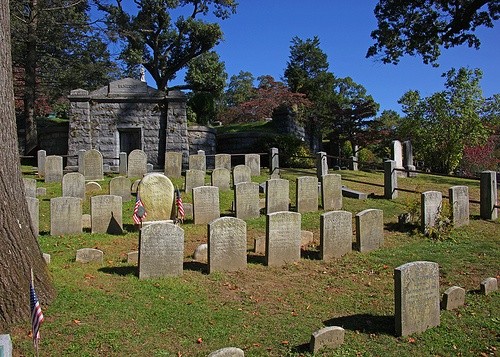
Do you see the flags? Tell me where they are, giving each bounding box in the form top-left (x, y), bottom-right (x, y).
top-left (27, 280), bottom-right (45, 350)
top-left (132, 190), bottom-right (147, 225)
top-left (177, 189), bottom-right (184, 220)
